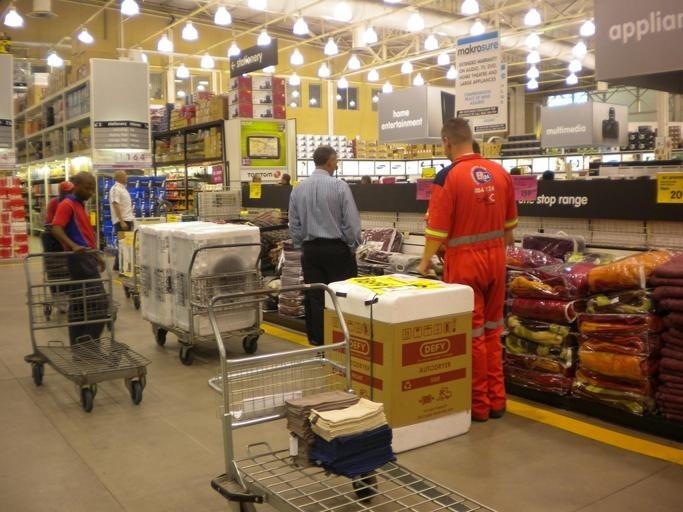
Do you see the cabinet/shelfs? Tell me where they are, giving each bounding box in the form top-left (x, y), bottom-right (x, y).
top-left (147, 118), bottom-right (296, 218)
top-left (15, 58), bottom-right (153, 252)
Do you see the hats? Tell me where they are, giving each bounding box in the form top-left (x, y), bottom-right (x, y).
top-left (58, 181), bottom-right (74, 193)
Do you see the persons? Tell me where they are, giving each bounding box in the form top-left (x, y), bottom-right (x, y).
top-left (52, 171), bottom-right (109, 362)
top-left (413, 115), bottom-right (519, 423)
top-left (41, 180), bottom-right (74, 314)
top-left (360, 175), bottom-right (371, 185)
top-left (288, 145), bottom-right (362, 360)
top-left (282, 173), bottom-right (291, 185)
top-left (251, 173), bottom-right (263, 183)
top-left (110, 170), bottom-right (133, 272)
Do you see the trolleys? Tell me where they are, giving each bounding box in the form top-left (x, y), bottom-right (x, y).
top-left (210, 283), bottom-right (498, 511)
top-left (24, 249), bottom-right (152, 413)
top-left (117, 230), bottom-right (142, 310)
top-left (142, 241), bottom-right (264, 366)
top-left (41, 228), bottom-right (121, 322)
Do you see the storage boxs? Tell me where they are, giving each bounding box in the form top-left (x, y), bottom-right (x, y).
top-left (169, 225), bottom-right (261, 334)
top-left (227, 76), bottom-right (286, 94)
top-left (227, 104), bottom-right (287, 119)
top-left (324, 274), bottom-right (478, 453)
top-left (227, 89), bottom-right (287, 106)
top-left (139, 222), bottom-right (218, 326)
